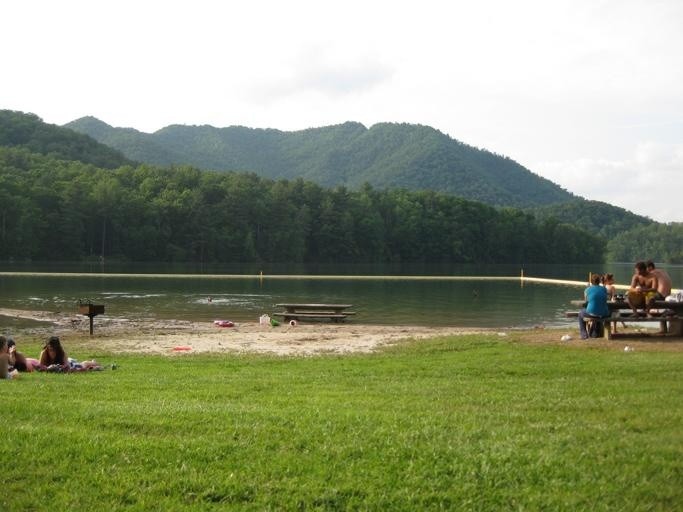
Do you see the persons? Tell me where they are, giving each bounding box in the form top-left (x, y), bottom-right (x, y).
top-left (645, 260), bottom-right (672, 312)
top-left (6, 338), bottom-right (39, 372)
top-left (625, 260), bottom-right (658, 317)
top-left (68, 357), bottom-right (97, 371)
top-left (40, 336), bottom-right (69, 372)
top-left (0, 335), bottom-right (19, 379)
top-left (601, 272), bottom-right (628, 333)
top-left (578, 273), bottom-right (610, 340)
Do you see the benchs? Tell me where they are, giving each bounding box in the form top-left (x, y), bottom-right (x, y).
top-left (272, 300), bottom-right (357, 323)
top-left (564, 297), bottom-right (683, 340)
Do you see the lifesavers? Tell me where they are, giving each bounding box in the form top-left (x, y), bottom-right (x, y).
top-left (215, 321), bottom-right (234, 326)
